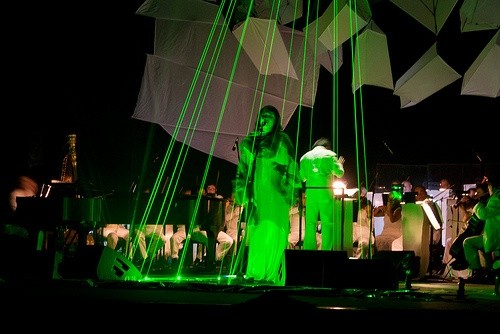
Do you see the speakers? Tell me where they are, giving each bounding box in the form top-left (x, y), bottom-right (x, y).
top-left (284, 249), bottom-right (421, 290)
top-left (58, 245), bottom-right (144, 282)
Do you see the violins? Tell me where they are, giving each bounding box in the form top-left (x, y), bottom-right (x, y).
top-left (453, 193), bottom-right (490, 210)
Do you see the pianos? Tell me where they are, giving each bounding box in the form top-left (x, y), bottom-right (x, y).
top-left (10, 181), bottom-right (227, 277)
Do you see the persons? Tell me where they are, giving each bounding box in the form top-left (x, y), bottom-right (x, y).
top-left (234, 106), bottom-right (302, 282)
top-left (300, 138), bottom-right (345, 250)
top-left (103, 179), bottom-right (500, 285)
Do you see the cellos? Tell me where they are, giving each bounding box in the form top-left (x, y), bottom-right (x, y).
top-left (441, 212), bottom-right (486, 283)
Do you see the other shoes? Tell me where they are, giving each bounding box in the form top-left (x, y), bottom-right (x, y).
top-left (468, 270), bottom-right (494, 283)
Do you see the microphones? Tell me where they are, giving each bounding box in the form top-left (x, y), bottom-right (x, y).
top-left (232, 137), bottom-right (238, 151)
top-left (260, 121), bottom-right (267, 129)
